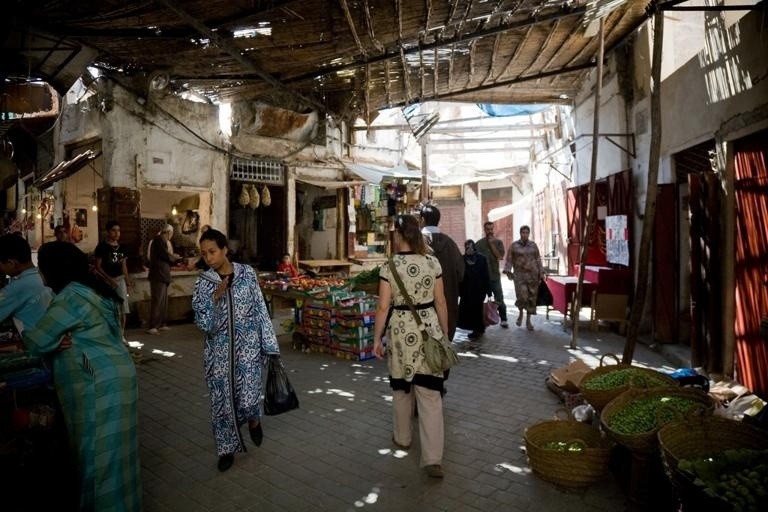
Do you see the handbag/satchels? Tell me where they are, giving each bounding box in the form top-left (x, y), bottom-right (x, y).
top-left (483, 296), bottom-right (500, 327)
top-left (421, 330), bottom-right (460, 375)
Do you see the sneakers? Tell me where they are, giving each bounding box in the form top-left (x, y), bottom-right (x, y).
top-left (526, 320), bottom-right (534, 331)
top-left (516, 315), bottom-right (523, 326)
top-left (467, 328), bottom-right (486, 340)
top-left (392, 435), bottom-right (412, 449)
top-left (422, 465), bottom-right (445, 478)
top-left (500, 320), bottom-right (508, 328)
top-left (159, 326), bottom-right (171, 331)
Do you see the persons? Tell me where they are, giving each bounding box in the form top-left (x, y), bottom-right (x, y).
top-left (277, 253), bottom-right (297, 276)
top-left (373, 216), bottom-right (460, 477)
top-left (502, 226), bottom-right (554, 331)
top-left (146, 230), bottom-right (174, 261)
top-left (475, 222), bottom-right (508, 328)
top-left (419, 204), bottom-right (466, 395)
top-left (0, 231), bottom-right (56, 341)
top-left (146, 224), bottom-right (183, 335)
top-left (187, 225), bottom-right (211, 272)
top-left (55, 225), bottom-right (67, 241)
top-left (192, 230), bottom-right (300, 472)
top-left (21, 240), bottom-right (144, 512)
top-left (457, 239), bottom-right (490, 339)
top-left (95, 221), bottom-right (134, 348)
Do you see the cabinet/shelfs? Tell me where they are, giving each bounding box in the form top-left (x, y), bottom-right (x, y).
top-left (539, 256), bottom-right (560, 279)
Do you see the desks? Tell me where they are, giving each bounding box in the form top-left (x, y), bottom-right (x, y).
top-left (545, 275), bottom-right (597, 334)
top-left (298, 259), bottom-right (354, 279)
top-left (260, 283), bottom-right (309, 350)
top-left (581, 265), bottom-right (629, 329)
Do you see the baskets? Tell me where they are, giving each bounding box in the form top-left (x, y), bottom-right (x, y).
top-left (655, 406), bottom-right (768, 512)
top-left (521, 406), bottom-right (617, 494)
top-left (598, 374), bottom-right (716, 455)
top-left (579, 352), bottom-right (681, 422)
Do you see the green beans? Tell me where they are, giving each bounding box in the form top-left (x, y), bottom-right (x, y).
top-left (580, 368), bottom-right (706, 435)
top-left (541, 439), bottom-right (584, 452)
top-left (680, 447), bottom-right (768, 512)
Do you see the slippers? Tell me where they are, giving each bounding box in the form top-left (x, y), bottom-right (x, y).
top-left (145, 328), bottom-right (160, 336)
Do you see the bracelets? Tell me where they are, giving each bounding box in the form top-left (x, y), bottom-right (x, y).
top-left (213, 291), bottom-right (220, 301)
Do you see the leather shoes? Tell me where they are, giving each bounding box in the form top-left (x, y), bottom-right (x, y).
top-left (248, 418), bottom-right (264, 448)
top-left (217, 453), bottom-right (234, 473)
